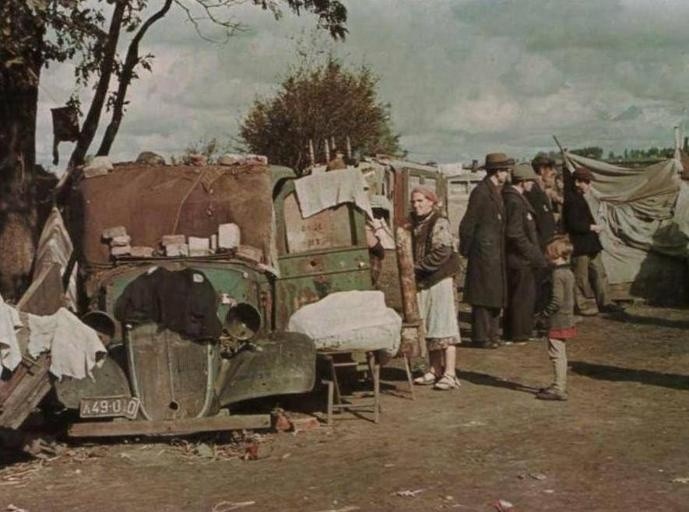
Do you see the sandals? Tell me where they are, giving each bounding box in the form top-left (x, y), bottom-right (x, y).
top-left (434, 374), bottom-right (461, 390)
top-left (413, 371), bottom-right (442, 385)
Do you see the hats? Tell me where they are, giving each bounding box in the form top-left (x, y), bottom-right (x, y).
top-left (510, 163), bottom-right (539, 183)
top-left (572, 168), bottom-right (596, 181)
top-left (478, 151), bottom-right (517, 170)
top-left (531, 156), bottom-right (555, 175)
top-left (410, 185), bottom-right (438, 203)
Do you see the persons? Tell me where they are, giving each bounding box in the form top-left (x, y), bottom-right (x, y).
top-left (405, 186), bottom-right (462, 391)
top-left (324, 146), bottom-right (361, 173)
top-left (460, 150), bottom-right (568, 349)
top-left (533, 234), bottom-right (577, 402)
top-left (563, 165), bottom-right (627, 315)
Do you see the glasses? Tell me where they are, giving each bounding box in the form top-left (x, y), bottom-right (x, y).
top-left (584, 180), bottom-right (590, 184)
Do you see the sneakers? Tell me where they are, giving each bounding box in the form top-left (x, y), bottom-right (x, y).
top-left (535, 386), bottom-right (570, 402)
top-left (571, 304), bottom-right (626, 322)
top-left (472, 335), bottom-right (546, 349)
